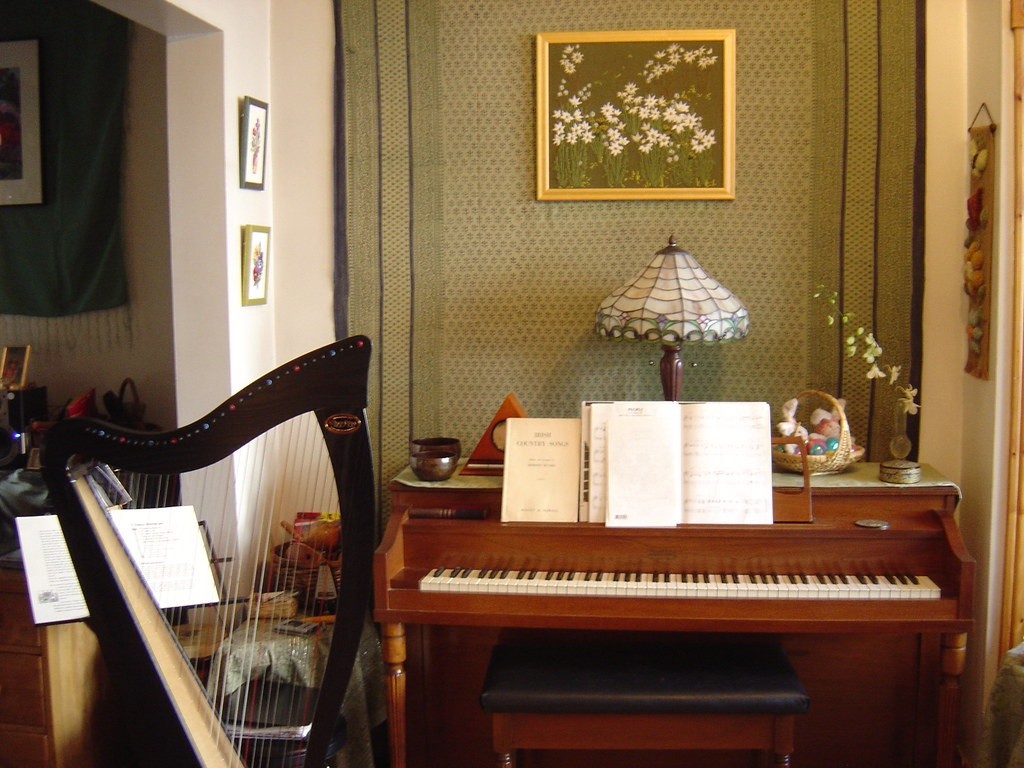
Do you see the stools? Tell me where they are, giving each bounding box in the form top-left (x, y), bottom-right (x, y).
top-left (224, 713), bottom-right (349, 768)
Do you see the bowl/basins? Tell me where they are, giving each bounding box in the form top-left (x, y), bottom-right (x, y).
top-left (410, 451), bottom-right (458, 481)
top-left (410, 437), bottom-right (462, 461)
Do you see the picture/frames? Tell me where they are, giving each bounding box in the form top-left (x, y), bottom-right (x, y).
top-left (535, 29), bottom-right (736, 201)
top-left (0, 345), bottom-right (32, 390)
top-left (242, 225), bottom-right (270, 308)
top-left (0, 38), bottom-right (45, 206)
top-left (240, 95), bottom-right (269, 192)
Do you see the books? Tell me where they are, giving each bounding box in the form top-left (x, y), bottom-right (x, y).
top-left (500, 417), bottom-right (581, 523)
top-left (580, 400), bottom-right (773, 529)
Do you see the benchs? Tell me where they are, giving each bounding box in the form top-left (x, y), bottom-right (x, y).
top-left (482, 640), bottom-right (808, 768)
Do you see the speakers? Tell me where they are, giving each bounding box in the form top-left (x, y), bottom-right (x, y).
top-left (0, 385), bottom-right (48, 471)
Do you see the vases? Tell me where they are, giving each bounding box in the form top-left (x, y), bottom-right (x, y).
top-left (890, 406), bottom-right (910, 457)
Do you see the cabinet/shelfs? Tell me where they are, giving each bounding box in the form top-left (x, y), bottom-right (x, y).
top-left (0, 567), bottom-right (115, 768)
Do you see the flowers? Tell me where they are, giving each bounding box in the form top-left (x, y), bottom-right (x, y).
top-left (815, 283), bottom-right (922, 416)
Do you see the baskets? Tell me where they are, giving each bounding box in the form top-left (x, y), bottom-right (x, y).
top-left (771, 390), bottom-right (865, 473)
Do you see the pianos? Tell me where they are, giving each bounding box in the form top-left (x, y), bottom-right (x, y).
top-left (373, 460), bottom-right (974, 768)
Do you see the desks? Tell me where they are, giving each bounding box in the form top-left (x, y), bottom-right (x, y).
top-left (206, 610), bottom-right (386, 768)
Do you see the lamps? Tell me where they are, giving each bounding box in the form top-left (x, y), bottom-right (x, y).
top-left (596, 235), bottom-right (747, 401)
